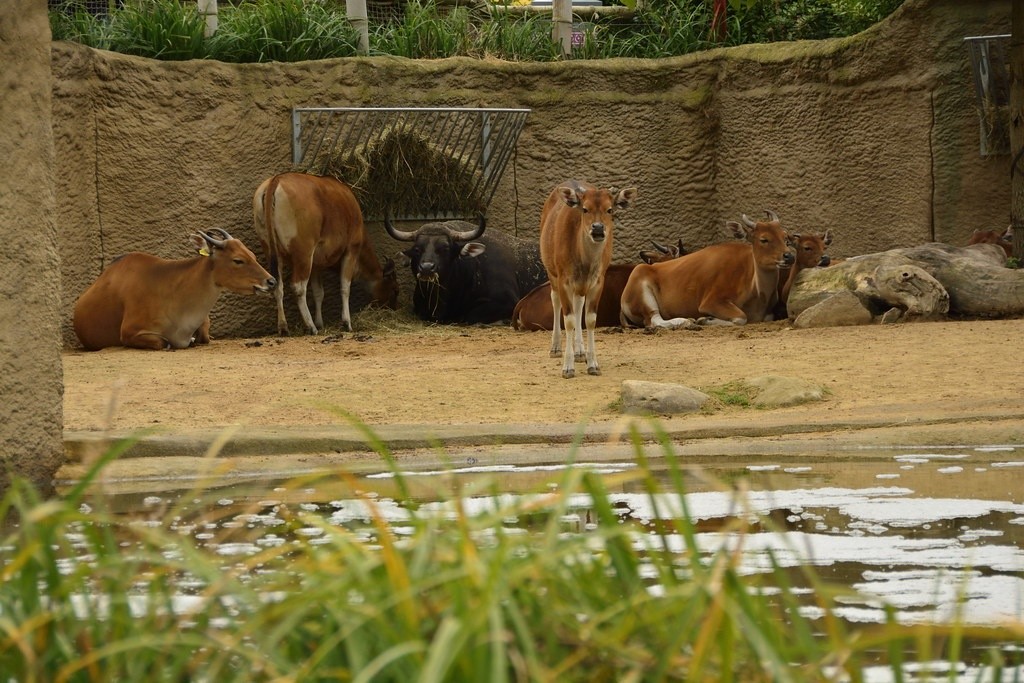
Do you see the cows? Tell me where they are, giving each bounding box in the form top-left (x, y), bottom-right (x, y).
top-left (74, 228), bottom-right (278, 351)
top-left (384, 199), bottom-right (550, 326)
top-left (513, 209), bottom-right (846, 336)
top-left (540, 177), bottom-right (638, 379)
top-left (964, 225), bottom-right (1014, 258)
top-left (252, 174), bottom-right (400, 337)
